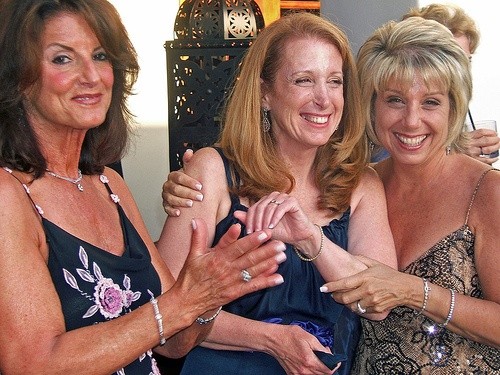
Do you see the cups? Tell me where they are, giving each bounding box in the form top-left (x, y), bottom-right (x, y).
top-left (465, 120), bottom-right (498, 158)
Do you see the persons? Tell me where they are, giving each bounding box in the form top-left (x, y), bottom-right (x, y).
top-left (0, 0), bottom-right (287, 375)
top-left (160, 16), bottom-right (500, 375)
top-left (155, 11), bottom-right (398, 375)
top-left (371, 4), bottom-right (500, 164)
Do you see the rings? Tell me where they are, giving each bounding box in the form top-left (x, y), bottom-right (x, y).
top-left (241, 269), bottom-right (251, 282)
top-left (356, 302), bottom-right (366, 315)
top-left (480, 146), bottom-right (483, 155)
top-left (269, 199), bottom-right (280, 206)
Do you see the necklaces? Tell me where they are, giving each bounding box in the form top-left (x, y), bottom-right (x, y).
top-left (45, 169), bottom-right (84, 191)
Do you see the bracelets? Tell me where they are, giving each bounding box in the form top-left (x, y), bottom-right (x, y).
top-left (196, 306), bottom-right (222, 326)
top-left (437, 288), bottom-right (455, 328)
top-left (294, 223), bottom-right (324, 261)
top-left (414, 277), bottom-right (431, 317)
top-left (147, 288), bottom-right (166, 346)
